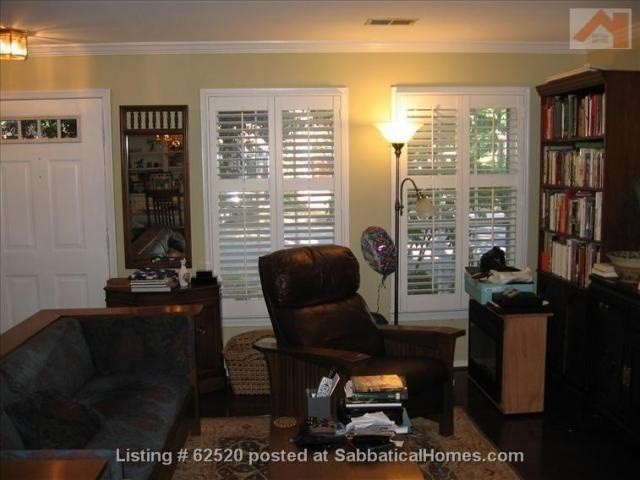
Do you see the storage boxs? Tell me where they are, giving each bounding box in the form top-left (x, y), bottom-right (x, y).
top-left (462, 263), bottom-right (538, 308)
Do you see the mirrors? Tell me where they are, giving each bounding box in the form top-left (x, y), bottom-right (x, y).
top-left (116, 101), bottom-right (193, 273)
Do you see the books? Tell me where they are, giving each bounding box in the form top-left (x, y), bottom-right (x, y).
top-left (537, 92), bottom-right (620, 293)
top-left (340, 372), bottom-right (411, 453)
top-left (129, 265), bottom-right (181, 293)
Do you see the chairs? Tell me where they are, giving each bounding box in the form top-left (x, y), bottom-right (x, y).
top-left (145, 187), bottom-right (182, 236)
top-left (253, 242), bottom-right (469, 441)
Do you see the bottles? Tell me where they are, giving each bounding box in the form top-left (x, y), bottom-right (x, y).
top-left (179, 259), bottom-right (190, 287)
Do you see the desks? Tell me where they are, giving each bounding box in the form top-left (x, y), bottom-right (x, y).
top-left (262, 401), bottom-right (421, 480)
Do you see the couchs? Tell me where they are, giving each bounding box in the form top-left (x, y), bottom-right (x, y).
top-left (126, 227), bottom-right (185, 270)
top-left (0, 301), bottom-right (204, 479)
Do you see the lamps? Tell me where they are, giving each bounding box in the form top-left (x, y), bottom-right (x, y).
top-left (0, 23), bottom-right (37, 64)
top-left (375, 116), bottom-right (436, 326)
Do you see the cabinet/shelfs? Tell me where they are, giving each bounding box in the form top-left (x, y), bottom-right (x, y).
top-left (533, 66), bottom-right (639, 422)
top-left (579, 270), bottom-right (639, 446)
top-left (127, 138), bottom-right (184, 227)
top-left (100, 275), bottom-right (225, 419)
top-left (466, 296), bottom-right (557, 419)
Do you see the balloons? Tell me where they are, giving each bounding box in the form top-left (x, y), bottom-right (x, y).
top-left (361, 224), bottom-right (397, 283)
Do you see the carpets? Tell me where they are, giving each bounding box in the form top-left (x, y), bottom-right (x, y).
top-left (171, 399), bottom-right (523, 480)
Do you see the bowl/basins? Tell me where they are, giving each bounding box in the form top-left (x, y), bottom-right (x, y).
top-left (606, 249), bottom-right (640, 281)
